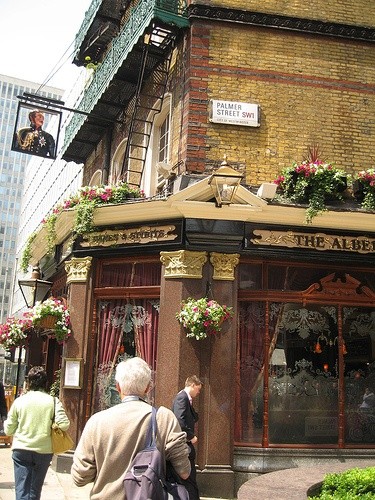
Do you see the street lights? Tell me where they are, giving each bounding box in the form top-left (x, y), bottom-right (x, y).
top-left (17, 279), bottom-right (54, 380)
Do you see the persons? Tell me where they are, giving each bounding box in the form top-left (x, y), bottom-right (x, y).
top-left (173, 374), bottom-right (205, 500)
top-left (71, 357), bottom-right (191, 500)
top-left (14, 110), bottom-right (55, 158)
top-left (4, 365), bottom-right (70, 500)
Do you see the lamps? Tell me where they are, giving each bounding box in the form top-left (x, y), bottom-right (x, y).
top-left (17, 261), bottom-right (54, 307)
top-left (208, 154), bottom-right (242, 204)
top-left (258, 183), bottom-right (277, 201)
top-left (157, 163), bottom-right (175, 180)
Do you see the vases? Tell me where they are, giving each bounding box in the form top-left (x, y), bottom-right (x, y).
top-left (281, 178), bottom-right (345, 200)
top-left (190, 316), bottom-right (213, 335)
top-left (354, 176), bottom-right (375, 201)
top-left (37, 313), bottom-right (59, 330)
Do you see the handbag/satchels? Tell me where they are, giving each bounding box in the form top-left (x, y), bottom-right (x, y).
top-left (51, 397), bottom-right (74, 453)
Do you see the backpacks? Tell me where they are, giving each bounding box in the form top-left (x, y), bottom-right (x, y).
top-left (124, 406), bottom-right (199, 500)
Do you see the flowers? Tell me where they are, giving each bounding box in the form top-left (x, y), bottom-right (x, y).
top-left (23, 184), bottom-right (145, 262)
top-left (176, 298), bottom-right (232, 341)
top-left (0, 299), bottom-right (70, 351)
top-left (353, 169), bottom-right (375, 206)
top-left (273, 157), bottom-right (346, 222)
